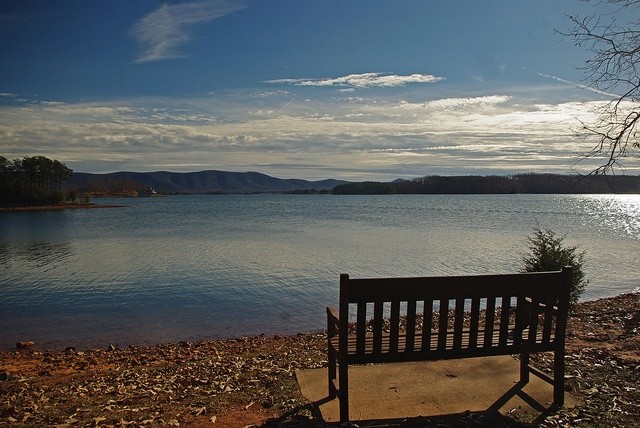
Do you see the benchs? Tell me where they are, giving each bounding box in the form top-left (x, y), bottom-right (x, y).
top-left (326, 264), bottom-right (574, 427)
